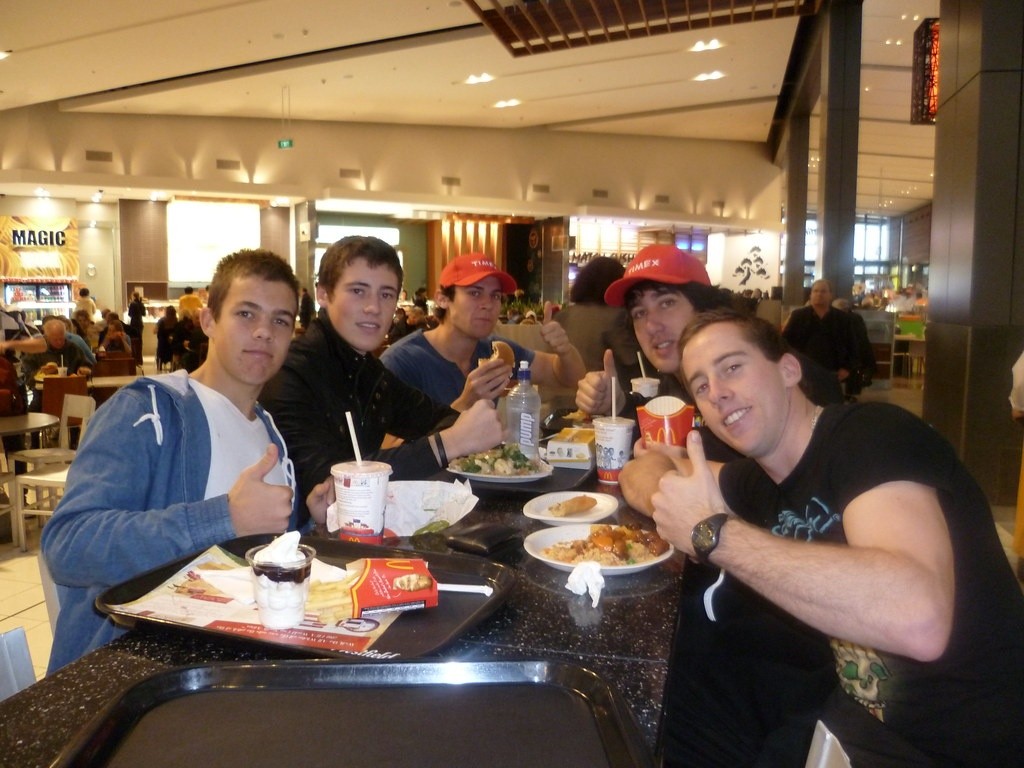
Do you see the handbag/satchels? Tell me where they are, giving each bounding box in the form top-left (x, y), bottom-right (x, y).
top-left (0, 357), bottom-right (24, 417)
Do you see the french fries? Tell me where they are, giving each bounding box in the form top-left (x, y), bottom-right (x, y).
top-left (306, 571), bottom-right (362, 623)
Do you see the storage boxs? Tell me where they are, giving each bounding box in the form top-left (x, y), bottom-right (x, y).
top-left (545, 427), bottom-right (599, 471)
top-left (345, 559), bottom-right (438, 619)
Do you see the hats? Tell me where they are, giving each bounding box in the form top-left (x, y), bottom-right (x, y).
top-left (605, 244), bottom-right (710, 307)
top-left (440, 252), bottom-right (517, 294)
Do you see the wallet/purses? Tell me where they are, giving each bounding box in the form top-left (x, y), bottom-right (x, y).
top-left (448, 522), bottom-right (523, 558)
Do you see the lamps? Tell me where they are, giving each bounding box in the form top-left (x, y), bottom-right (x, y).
top-left (910, 16), bottom-right (939, 125)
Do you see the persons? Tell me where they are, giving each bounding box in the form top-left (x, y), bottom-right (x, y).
top-left (298, 287), bottom-right (316, 327)
top-left (377, 254), bottom-right (586, 449)
top-left (388, 288), bottom-right (444, 349)
top-left (40, 247), bottom-right (335, 677)
top-left (0, 287), bottom-right (210, 518)
top-left (256, 237), bottom-right (502, 521)
top-left (618, 307), bottom-right (1024, 768)
top-left (506, 243), bottom-right (1024, 557)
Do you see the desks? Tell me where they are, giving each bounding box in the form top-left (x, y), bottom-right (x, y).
top-left (893, 331), bottom-right (927, 387)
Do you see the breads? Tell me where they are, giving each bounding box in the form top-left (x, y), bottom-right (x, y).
top-left (477, 340), bottom-right (516, 371)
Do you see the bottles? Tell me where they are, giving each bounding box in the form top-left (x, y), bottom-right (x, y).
top-left (505, 360), bottom-right (541, 461)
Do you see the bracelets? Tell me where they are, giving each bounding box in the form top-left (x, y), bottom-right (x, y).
top-left (435, 431), bottom-right (450, 470)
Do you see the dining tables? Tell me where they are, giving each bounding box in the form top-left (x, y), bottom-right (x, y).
top-left (1, 412), bottom-right (60, 500)
top-left (0, 415), bottom-right (689, 768)
top-left (34, 372), bottom-right (143, 390)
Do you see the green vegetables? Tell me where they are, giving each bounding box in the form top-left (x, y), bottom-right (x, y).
top-left (456, 446), bottom-right (534, 473)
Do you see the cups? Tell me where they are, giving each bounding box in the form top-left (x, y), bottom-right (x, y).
top-left (57, 367), bottom-right (68, 377)
top-left (330, 460), bottom-right (394, 546)
top-left (245, 540), bottom-right (316, 630)
top-left (592, 416), bottom-right (636, 487)
top-left (632, 379), bottom-right (661, 397)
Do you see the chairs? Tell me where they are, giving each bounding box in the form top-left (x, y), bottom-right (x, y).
top-left (92, 349), bottom-right (137, 377)
top-left (3, 393), bottom-right (96, 551)
top-left (38, 377), bottom-right (90, 450)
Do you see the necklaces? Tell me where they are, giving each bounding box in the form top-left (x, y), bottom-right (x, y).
top-left (812, 403), bottom-right (820, 435)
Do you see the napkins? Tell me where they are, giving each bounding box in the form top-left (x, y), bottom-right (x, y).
top-left (565, 559), bottom-right (604, 610)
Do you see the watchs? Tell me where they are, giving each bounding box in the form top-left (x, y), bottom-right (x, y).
top-left (691, 513), bottom-right (743, 569)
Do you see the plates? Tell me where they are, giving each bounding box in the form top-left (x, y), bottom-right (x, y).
top-left (523, 524), bottom-right (674, 576)
top-left (523, 491), bottom-right (620, 527)
top-left (446, 458), bottom-right (554, 483)
